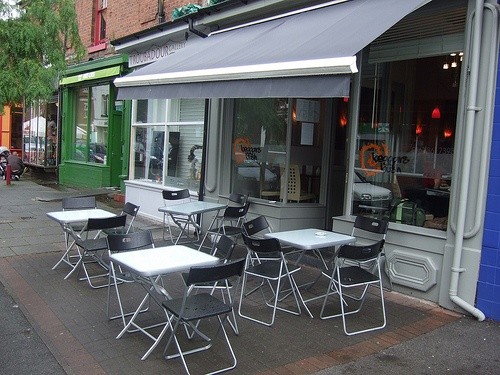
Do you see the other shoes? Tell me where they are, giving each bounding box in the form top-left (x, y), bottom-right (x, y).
top-left (15, 175), bottom-right (20, 181)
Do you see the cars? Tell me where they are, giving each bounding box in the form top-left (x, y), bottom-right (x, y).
top-left (232, 152), bottom-right (280, 198)
top-left (73, 142), bottom-right (107, 163)
top-left (24, 134), bottom-right (46, 152)
top-left (351, 169), bottom-right (394, 216)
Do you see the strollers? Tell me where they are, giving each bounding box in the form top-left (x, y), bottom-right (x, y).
top-left (0, 146), bottom-right (12, 180)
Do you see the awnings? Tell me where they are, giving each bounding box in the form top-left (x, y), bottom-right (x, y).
top-left (114, 0), bottom-right (434, 99)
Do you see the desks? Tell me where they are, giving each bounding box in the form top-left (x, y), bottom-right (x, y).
top-left (266, 166), bottom-right (321, 203)
top-left (108, 245), bottom-right (220, 360)
top-left (45, 209), bottom-right (117, 280)
top-left (157, 201), bottom-right (228, 251)
top-left (264, 227), bottom-right (356, 319)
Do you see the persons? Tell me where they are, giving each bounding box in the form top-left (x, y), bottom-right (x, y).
top-left (136, 135), bottom-right (143, 151)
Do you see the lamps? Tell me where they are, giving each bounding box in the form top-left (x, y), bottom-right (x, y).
top-left (431, 51), bottom-right (465, 118)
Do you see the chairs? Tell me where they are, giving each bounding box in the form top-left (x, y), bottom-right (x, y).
top-left (260, 164), bottom-right (317, 203)
top-left (62, 188), bottom-right (393, 375)
